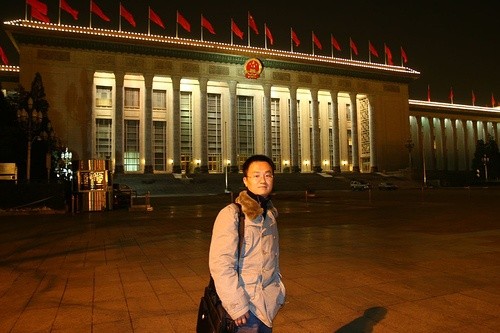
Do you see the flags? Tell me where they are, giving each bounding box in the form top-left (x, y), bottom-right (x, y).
top-left (90, 0), bottom-right (110, 21)
top-left (26, 0), bottom-right (50, 22)
top-left (202, 16), bottom-right (216, 34)
top-left (428, 87), bottom-right (496, 107)
top-left (266, 25), bottom-right (273, 44)
top-left (150, 8), bottom-right (165, 29)
top-left (120, 4), bottom-right (136, 28)
top-left (178, 12), bottom-right (190, 32)
top-left (292, 29), bottom-right (357, 56)
top-left (232, 20), bottom-right (244, 41)
top-left (249, 14), bottom-right (258, 35)
top-left (0, 46), bottom-right (9, 64)
top-left (60, 0), bottom-right (78, 20)
top-left (370, 42), bottom-right (407, 65)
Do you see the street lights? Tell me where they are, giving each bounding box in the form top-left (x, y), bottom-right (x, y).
top-left (17, 97), bottom-right (43, 181)
top-left (482, 154), bottom-right (490, 184)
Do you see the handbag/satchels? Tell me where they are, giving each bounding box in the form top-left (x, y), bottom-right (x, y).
top-left (197, 275), bottom-right (236, 333)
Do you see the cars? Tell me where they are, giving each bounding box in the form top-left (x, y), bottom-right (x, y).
top-left (378, 182), bottom-right (399, 192)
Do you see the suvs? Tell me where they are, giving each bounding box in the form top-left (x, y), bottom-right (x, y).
top-left (351, 180), bottom-right (374, 191)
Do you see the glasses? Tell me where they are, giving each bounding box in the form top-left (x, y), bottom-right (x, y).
top-left (247, 174), bottom-right (273, 180)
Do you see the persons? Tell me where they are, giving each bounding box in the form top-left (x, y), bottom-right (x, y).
top-left (209, 155), bottom-right (286, 333)
